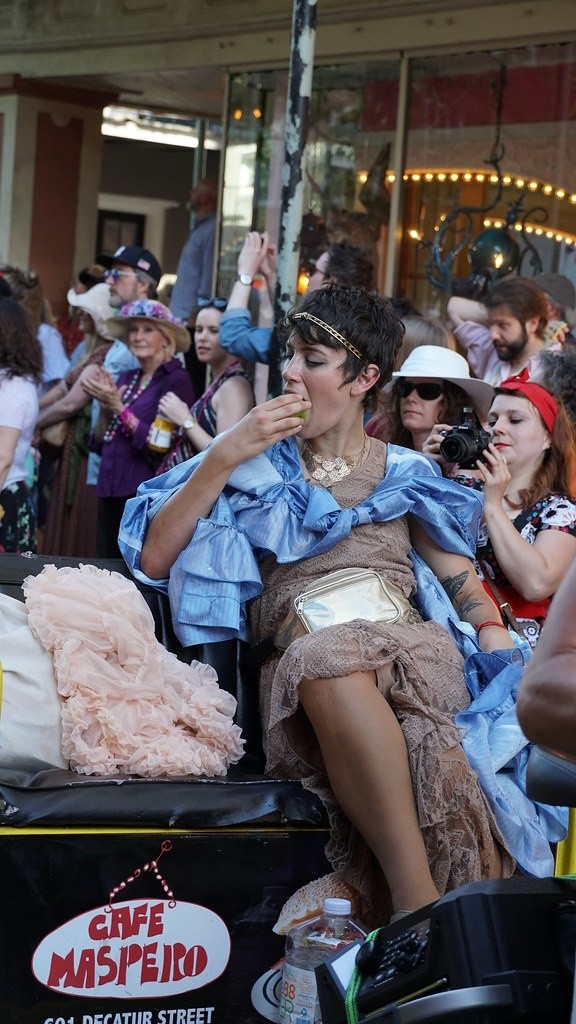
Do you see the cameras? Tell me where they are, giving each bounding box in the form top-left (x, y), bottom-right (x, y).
top-left (438, 421), bottom-right (491, 470)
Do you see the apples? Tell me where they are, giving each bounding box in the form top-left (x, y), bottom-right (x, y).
top-left (289, 411), bottom-right (308, 420)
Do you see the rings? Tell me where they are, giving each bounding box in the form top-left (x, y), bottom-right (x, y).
top-left (503, 457), bottom-right (507, 463)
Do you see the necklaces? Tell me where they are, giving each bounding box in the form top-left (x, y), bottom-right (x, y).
top-left (302, 431), bottom-right (368, 487)
top-left (104, 352), bottom-right (167, 441)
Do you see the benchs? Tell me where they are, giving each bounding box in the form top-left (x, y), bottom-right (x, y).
top-left (1, 556), bottom-right (334, 831)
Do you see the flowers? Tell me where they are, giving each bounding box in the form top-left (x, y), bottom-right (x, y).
top-left (121, 299), bottom-right (187, 327)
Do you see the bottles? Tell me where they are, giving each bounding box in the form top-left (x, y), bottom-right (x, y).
top-left (278, 897), bottom-right (366, 1023)
top-left (147, 409), bottom-right (174, 453)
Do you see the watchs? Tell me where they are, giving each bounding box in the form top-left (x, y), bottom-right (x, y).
top-left (183, 420), bottom-right (197, 432)
top-left (235, 273), bottom-right (254, 285)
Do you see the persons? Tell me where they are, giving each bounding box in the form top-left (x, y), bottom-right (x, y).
top-left (363, 300), bottom-right (494, 450)
top-left (146, 298), bottom-right (256, 477)
top-left (219, 233), bottom-right (377, 360)
top-left (0, 173), bottom-right (216, 558)
top-left (448, 278), bottom-right (568, 388)
top-left (424, 374), bottom-right (576, 639)
top-left (117, 285), bottom-right (572, 929)
top-left (516, 563), bottom-right (576, 758)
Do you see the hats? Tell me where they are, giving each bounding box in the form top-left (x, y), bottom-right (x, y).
top-left (96, 245), bottom-right (161, 285)
top-left (105, 298), bottom-right (191, 354)
top-left (525, 272), bottom-right (576, 307)
top-left (391, 345), bottom-right (495, 422)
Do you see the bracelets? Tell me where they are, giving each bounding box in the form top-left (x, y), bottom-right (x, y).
top-left (477, 622), bottom-right (507, 635)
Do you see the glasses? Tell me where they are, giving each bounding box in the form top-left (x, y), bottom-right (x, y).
top-left (197, 296), bottom-right (229, 311)
top-left (394, 380), bottom-right (451, 401)
top-left (101, 268), bottom-right (143, 282)
top-left (309, 263), bottom-right (332, 280)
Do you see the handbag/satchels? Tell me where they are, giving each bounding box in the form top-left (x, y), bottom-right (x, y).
top-left (290, 568), bottom-right (412, 649)
